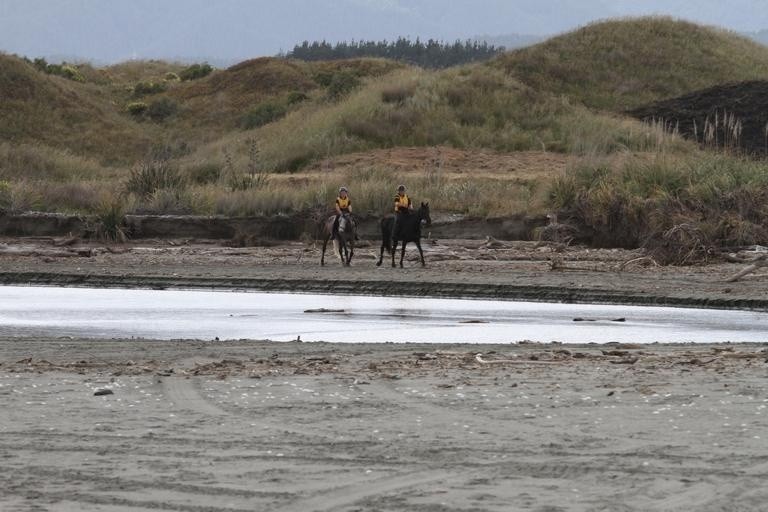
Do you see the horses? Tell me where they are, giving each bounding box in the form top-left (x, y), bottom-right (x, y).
top-left (375, 201), bottom-right (432, 268)
top-left (319, 210), bottom-right (358, 266)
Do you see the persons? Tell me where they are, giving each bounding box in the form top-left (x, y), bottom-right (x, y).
top-left (330, 187), bottom-right (359, 241)
top-left (392, 186), bottom-right (411, 241)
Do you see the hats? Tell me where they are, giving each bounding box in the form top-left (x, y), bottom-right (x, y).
top-left (397, 185), bottom-right (407, 192)
top-left (338, 186), bottom-right (347, 193)
